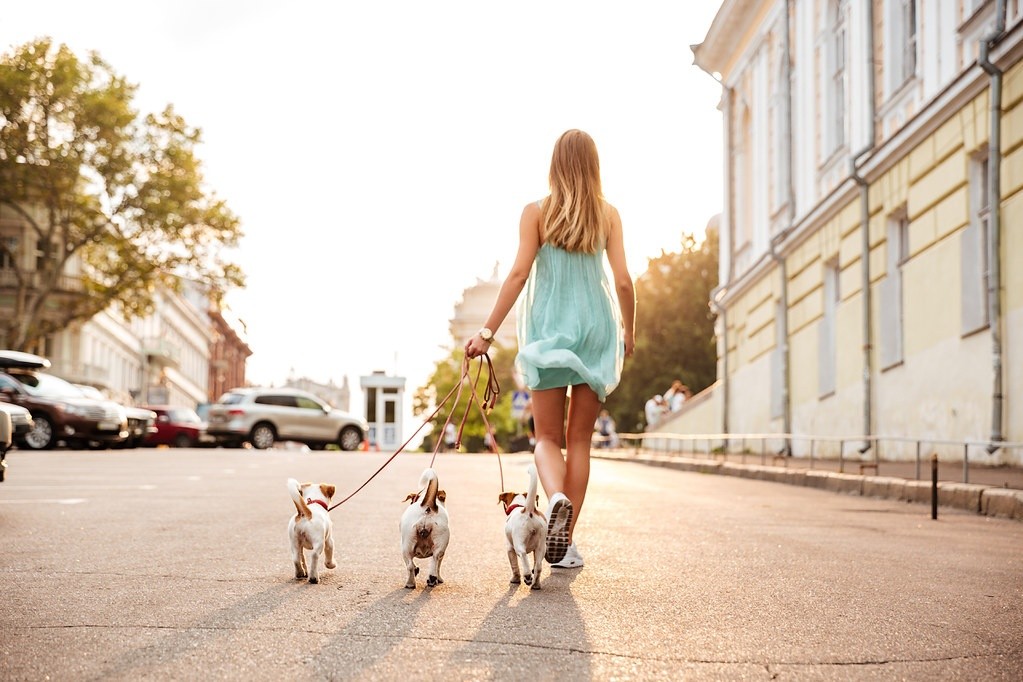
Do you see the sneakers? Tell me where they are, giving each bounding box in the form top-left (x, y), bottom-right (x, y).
top-left (551, 542), bottom-right (584, 567)
top-left (544, 493), bottom-right (575, 564)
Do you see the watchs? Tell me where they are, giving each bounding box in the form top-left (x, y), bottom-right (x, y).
top-left (479, 326), bottom-right (495, 344)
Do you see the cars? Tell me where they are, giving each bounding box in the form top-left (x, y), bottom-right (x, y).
top-left (73, 383), bottom-right (161, 449)
top-left (0, 349), bottom-right (130, 451)
top-left (205, 386), bottom-right (370, 452)
top-left (136, 405), bottom-right (213, 446)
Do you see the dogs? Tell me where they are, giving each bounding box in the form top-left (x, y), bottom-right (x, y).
top-left (496, 475), bottom-right (550, 590)
top-left (284, 476), bottom-right (337, 586)
top-left (398, 469), bottom-right (452, 589)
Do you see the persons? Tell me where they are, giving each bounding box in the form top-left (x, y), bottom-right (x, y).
top-left (465, 128), bottom-right (636, 569)
top-left (437, 377), bottom-right (696, 454)
